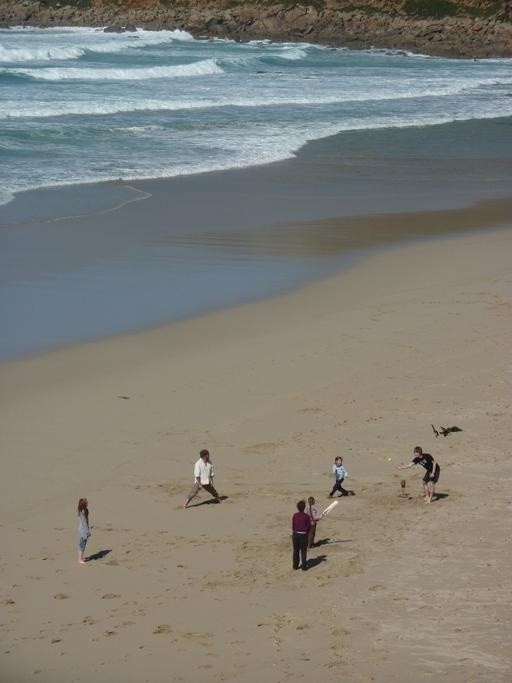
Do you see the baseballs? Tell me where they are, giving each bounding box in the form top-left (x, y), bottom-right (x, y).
top-left (388, 458), bottom-right (391, 461)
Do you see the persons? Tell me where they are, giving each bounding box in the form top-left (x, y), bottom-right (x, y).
top-left (328, 454), bottom-right (349, 499)
top-left (396, 446), bottom-right (440, 504)
top-left (184, 448), bottom-right (221, 509)
top-left (77, 497), bottom-right (91, 563)
top-left (304, 496), bottom-right (323, 548)
top-left (291, 500), bottom-right (311, 571)
